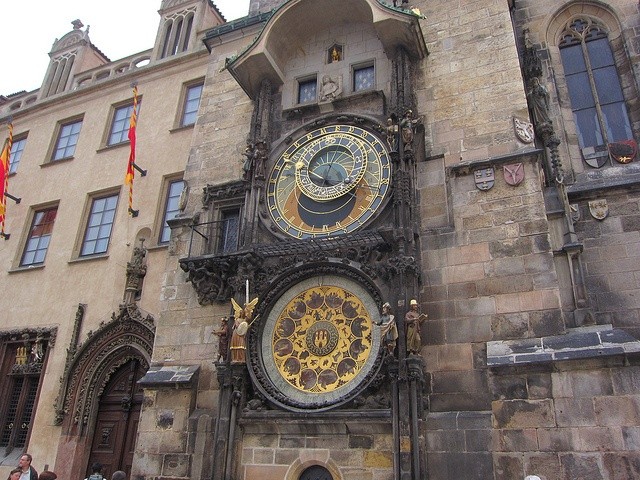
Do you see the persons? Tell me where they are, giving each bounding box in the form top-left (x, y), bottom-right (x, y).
top-left (254, 142), bottom-right (268, 180)
top-left (111, 471), bottom-right (126, 480)
top-left (320, 74), bottom-right (338, 101)
top-left (241, 146), bottom-right (253, 180)
top-left (39, 471), bottom-right (57, 480)
top-left (383, 118), bottom-right (398, 152)
top-left (83, 462), bottom-right (108, 480)
top-left (212, 317), bottom-right (229, 364)
top-left (7, 454), bottom-right (38, 480)
top-left (405, 299), bottom-right (427, 358)
top-left (7, 469), bottom-right (23, 480)
top-left (230, 297), bottom-right (259, 364)
top-left (371, 302), bottom-right (398, 360)
top-left (399, 109), bottom-right (425, 156)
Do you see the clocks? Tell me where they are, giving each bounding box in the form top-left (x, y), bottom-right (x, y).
top-left (245, 262), bottom-right (387, 416)
top-left (253, 112), bottom-right (394, 244)
